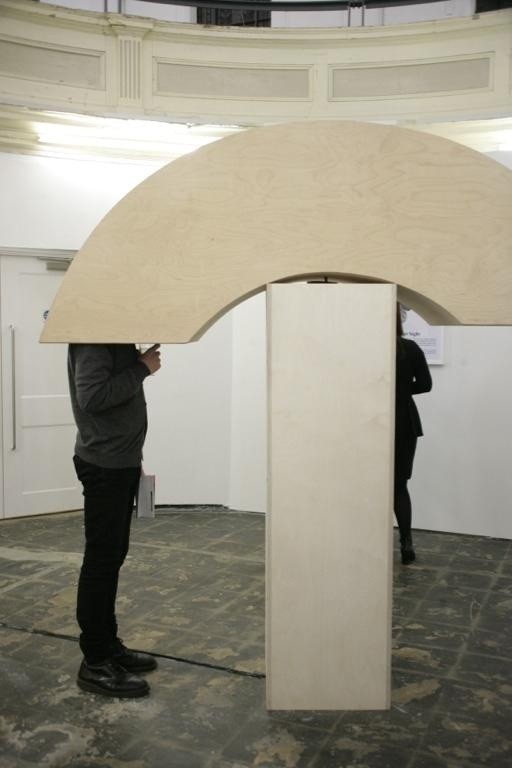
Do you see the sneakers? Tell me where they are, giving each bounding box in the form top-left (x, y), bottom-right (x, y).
top-left (399, 540), bottom-right (417, 566)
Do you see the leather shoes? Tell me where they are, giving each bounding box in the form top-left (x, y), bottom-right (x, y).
top-left (75, 655), bottom-right (153, 700)
top-left (110, 638), bottom-right (159, 674)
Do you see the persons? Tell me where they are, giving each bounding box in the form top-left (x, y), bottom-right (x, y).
top-left (67, 344), bottom-right (161, 698)
top-left (394, 301), bottom-right (432, 566)
